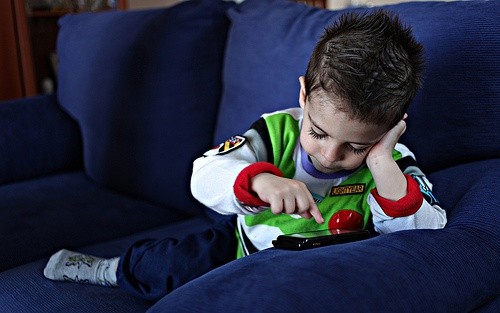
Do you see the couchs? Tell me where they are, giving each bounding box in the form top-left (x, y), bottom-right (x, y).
top-left (0, 0), bottom-right (500, 312)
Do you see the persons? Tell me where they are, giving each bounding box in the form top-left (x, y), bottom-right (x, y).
top-left (45, 8), bottom-right (446, 298)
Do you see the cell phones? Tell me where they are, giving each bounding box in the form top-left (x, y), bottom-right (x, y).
top-left (272, 227), bottom-right (360, 252)
